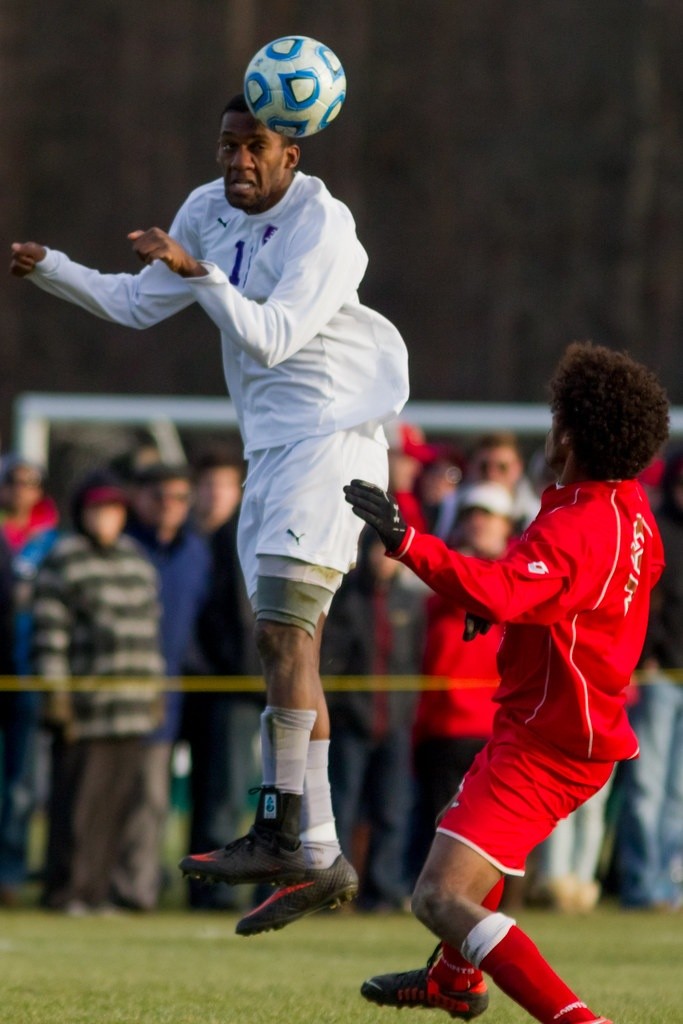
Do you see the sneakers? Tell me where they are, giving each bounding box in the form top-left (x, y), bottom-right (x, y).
top-left (180, 825), bottom-right (306, 887)
top-left (359, 941), bottom-right (489, 1022)
top-left (236, 854), bottom-right (360, 937)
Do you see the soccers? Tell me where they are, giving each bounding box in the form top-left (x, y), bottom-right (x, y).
top-left (242, 33), bottom-right (348, 140)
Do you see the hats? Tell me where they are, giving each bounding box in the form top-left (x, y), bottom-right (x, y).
top-left (458, 484), bottom-right (513, 521)
top-left (74, 470), bottom-right (124, 505)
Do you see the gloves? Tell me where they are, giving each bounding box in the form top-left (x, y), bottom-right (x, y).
top-left (463, 607), bottom-right (493, 641)
top-left (343, 480), bottom-right (408, 554)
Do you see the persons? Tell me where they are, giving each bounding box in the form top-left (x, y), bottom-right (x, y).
top-left (0, 433), bottom-right (683, 919)
top-left (342, 341), bottom-right (671, 1024)
top-left (13, 93), bottom-right (409, 938)
